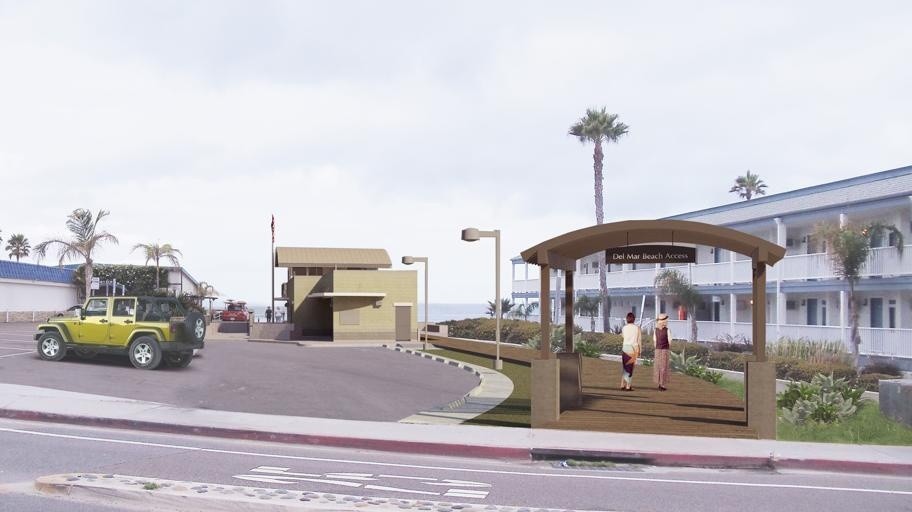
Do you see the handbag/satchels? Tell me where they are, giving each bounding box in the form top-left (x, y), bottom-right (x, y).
top-left (635, 344), bottom-right (643, 358)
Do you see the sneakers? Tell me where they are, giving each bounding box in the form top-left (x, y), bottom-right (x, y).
top-left (620, 385), bottom-right (634, 391)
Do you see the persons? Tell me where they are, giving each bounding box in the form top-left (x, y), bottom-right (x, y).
top-left (653, 314), bottom-right (670, 390)
top-left (265, 306), bottom-right (272, 322)
top-left (275, 306), bottom-right (282, 322)
top-left (619, 312), bottom-right (643, 390)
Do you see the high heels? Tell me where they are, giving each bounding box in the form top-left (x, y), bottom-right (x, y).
top-left (655, 384), bottom-right (667, 392)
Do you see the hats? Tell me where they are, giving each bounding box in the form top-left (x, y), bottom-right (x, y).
top-left (655, 313), bottom-right (669, 322)
